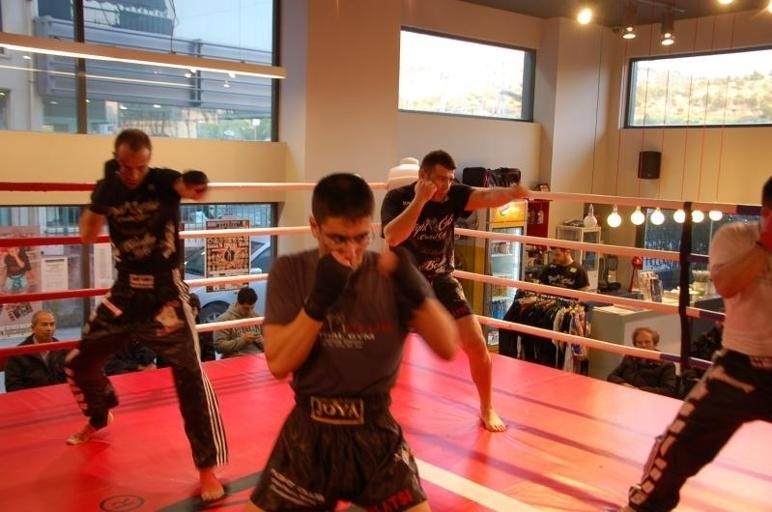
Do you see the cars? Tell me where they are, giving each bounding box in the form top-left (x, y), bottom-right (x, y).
top-left (181, 234), bottom-right (274, 324)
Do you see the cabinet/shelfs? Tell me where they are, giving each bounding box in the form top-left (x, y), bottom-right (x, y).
top-left (556, 224), bottom-right (601, 291)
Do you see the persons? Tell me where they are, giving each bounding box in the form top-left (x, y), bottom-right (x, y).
top-left (619, 177), bottom-right (772, 512)
top-left (64, 128), bottom-right (232, 504)
top-left (608, 327), bottom-right (679, 396)
top-left (249, 173), bottom-right (462, 511)
top-left (541, 246), bottom-right (591, 315)
top-left (6, 309), bottom-right (72, 394)
top-left (157, 292), bottom-right (214, 369)
top-left (683, 306), bottom-right (727, 391)
top-left (379, 148), bottom-right (535, 433)
top-left (2, 235), bottom-right (33, 320)
top-left (214, 287), bottom-right (267, 361)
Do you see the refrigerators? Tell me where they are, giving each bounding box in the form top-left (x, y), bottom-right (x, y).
top-left (451, 195), bottom-right (528, 358)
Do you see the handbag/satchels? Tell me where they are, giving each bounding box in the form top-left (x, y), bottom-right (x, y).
top-left (463, 165), bottom-right (522, 188)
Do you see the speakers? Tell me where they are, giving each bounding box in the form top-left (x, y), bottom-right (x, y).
top-left (638, 151), bottom-right (661, 179)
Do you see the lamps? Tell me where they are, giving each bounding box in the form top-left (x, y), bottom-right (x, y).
top-left (583, 204), bottom-right (666, 228)
top-left (623, 1), bottom-right (685, 46)
